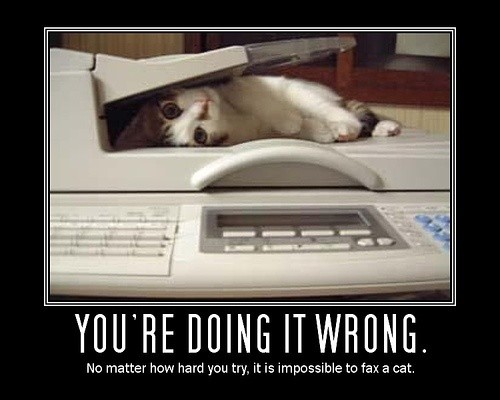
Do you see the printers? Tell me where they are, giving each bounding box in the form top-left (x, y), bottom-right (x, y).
top-left (49, 35), bottom-right (450, 301)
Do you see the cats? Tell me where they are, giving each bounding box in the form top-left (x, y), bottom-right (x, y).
top-left (118, 72), bottom-right (401, 149)
